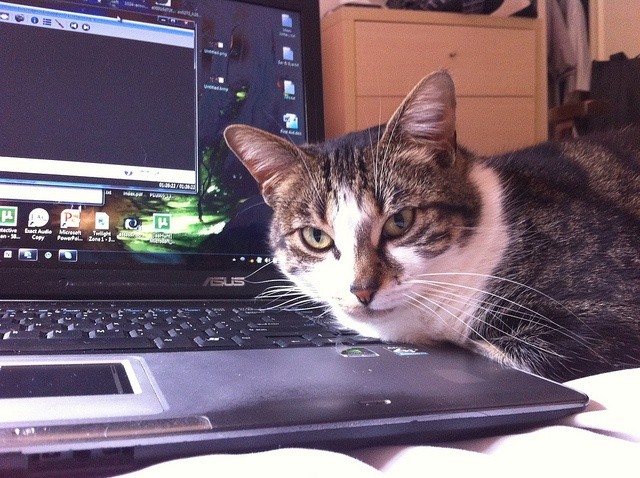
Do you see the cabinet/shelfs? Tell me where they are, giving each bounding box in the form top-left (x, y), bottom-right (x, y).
top-left (320, 5), bottom-right (550, 158)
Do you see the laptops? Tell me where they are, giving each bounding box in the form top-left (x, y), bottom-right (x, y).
top-left (0, 0), bottom-right (591, 477)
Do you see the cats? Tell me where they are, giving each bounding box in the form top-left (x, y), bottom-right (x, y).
top-left (221, 66), bottom-right (640, 386)
